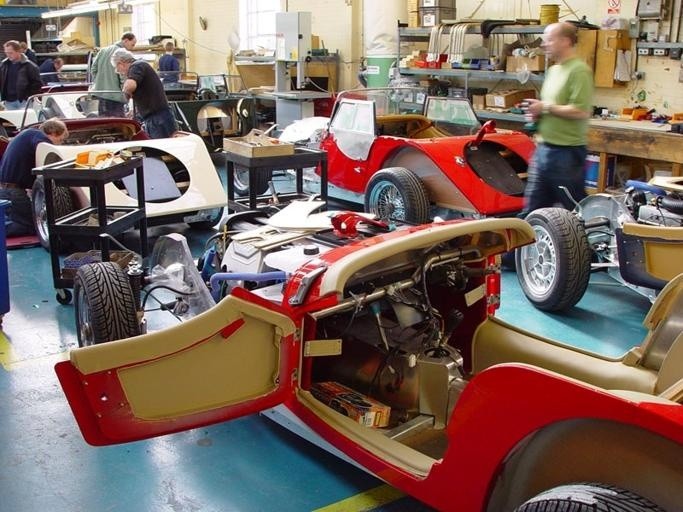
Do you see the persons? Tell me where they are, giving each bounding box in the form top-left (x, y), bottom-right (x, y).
top-left (36, 58), bottom-right (66, 86)
top-left (0, 117), bottom-right (70, 239)
top-left (108, 47), bottom-right (177, 139)
top-left (491, 21), bottom-right (597, 273)
top-left (157, 40), bottom-right (181, 83)
top-left (0, 39), bottom-right (44, 103)
top-left (17, 42), bottom-right (39, 66)
top-left (88, 33), bottom-right (137, 118)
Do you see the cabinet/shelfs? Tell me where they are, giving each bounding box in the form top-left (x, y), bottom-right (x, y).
top-left (226, 147), bottom-right (328, 216)
top-left (395, 20), bottom-right (549, 138)
top-left (32, 153), bottom-right (149, 306)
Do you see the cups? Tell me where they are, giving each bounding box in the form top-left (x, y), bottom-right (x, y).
top-left (120, 149), bottom-right (132, 162)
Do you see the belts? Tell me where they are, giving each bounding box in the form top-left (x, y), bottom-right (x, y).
top-left (0, 183), bottom-right (24, 189)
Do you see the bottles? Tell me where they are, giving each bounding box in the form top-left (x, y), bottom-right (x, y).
top-left (639, 31), bottom-right (656, 42)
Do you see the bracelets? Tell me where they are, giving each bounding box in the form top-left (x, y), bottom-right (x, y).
top-left (541, 102), bottom-right (552, 115)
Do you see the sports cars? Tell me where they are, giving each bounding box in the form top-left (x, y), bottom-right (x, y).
top-left (0, 48), bottom-right (260, 246)
top-left (47, 210), bottom-right (683, 512)
top-left (514, 169), bottom-right (683, 313)
top-left (229, 67), bottom-right (548, 233)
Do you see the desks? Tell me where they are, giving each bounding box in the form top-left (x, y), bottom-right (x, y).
top-left (584, 112), bottom-right (683, 193)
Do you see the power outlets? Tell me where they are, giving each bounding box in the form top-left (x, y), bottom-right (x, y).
top-left (635, 71), bottom-right (645, 81)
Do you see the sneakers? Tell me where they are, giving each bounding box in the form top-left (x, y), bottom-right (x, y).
top-left (500, 253), bottom-right (515, 271)
